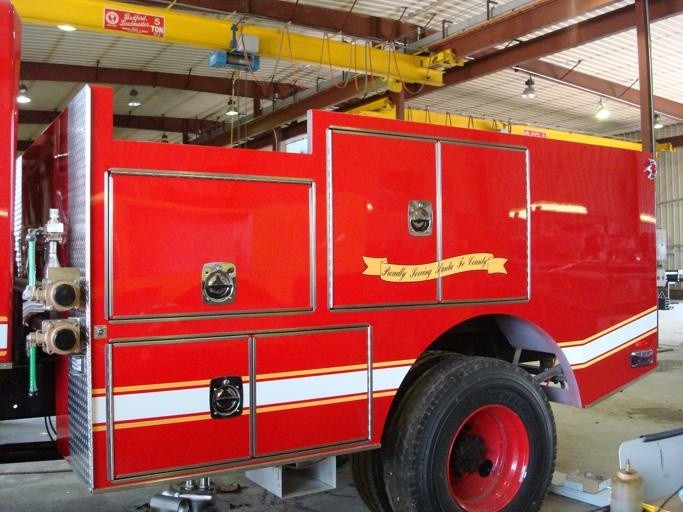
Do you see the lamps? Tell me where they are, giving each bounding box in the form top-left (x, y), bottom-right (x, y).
top-left (14, 79), bottom-right (241, 117)
top-left (521, 74), bottom-right (664, 130)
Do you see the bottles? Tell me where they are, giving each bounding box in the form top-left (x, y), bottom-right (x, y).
top-left (610, 459), bottom-right (643, 512)
top-left (47, 208), bottom-right (60, 239)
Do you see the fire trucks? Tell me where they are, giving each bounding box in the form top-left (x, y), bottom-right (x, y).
top-left (0, 0), bottom-right (660, 512)
